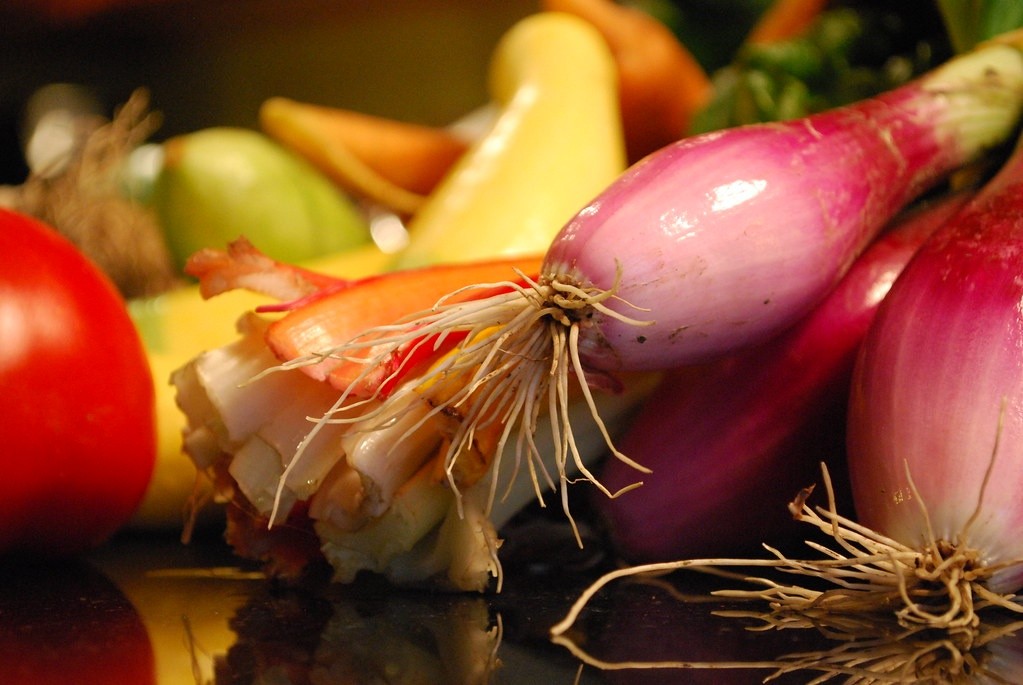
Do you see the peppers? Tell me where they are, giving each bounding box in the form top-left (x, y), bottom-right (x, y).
top-left (99, 0), bottom-right (849, 529)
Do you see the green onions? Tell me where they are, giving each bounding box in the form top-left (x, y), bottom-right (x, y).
top-left (540, 0), bottom-right (1023, 662)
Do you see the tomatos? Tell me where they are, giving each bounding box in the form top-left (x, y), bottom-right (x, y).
top-left (0, 206), bottom-right (160, 558)
